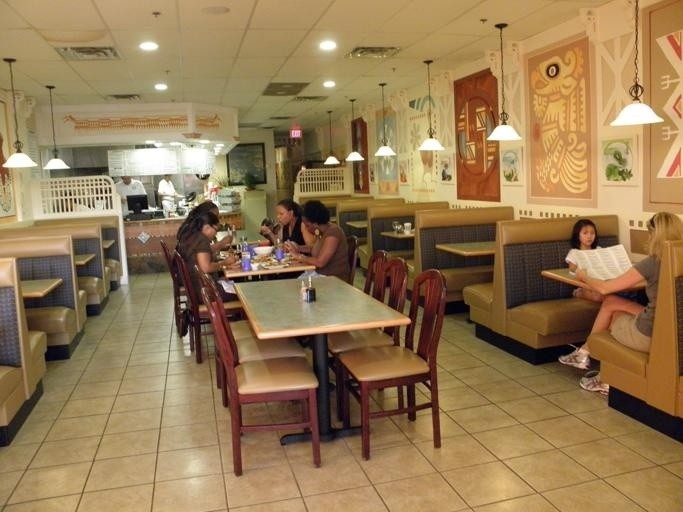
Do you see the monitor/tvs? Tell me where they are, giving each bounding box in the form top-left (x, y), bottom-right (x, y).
top-left (127, 195), bottom-right (148, 214)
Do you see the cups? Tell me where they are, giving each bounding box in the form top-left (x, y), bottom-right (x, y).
top-left (392, 221), bottom-right (412, 235)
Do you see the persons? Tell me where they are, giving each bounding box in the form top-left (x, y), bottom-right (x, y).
top-left (157, 175), bottom-right (180, 218)
top-left (258, 199), bottom-right (317, 278)
top-left (558, 212), bottom-right (683, 396)
top-left (115, 177), bottom-right (147, 214)
top-left (176, 211), bottom-right (240, 321)
top-left (172, 200), bottom-right (232, 286)
top-left (564, 219), bottom-right (615, 303)
top-left (283, 200), bottom-right (351, 283)
top-left (62, 192), bottom-right (92, 213)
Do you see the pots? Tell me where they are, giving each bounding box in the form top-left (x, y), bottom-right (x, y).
top-left (184, 191), bottom-right (196, 202)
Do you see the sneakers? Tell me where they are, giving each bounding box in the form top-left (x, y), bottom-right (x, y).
top-left (558, 347), bottom-right (591, 370)
top-left (579, 373), bottom-right (609, 392)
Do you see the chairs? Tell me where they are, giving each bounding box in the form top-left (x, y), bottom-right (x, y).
top-left (173, 249), bottom-right (244, 364)
top-left (204, 272), bottom-right (308, 435)
top-left (194, 264), bottom-right (258, 408)
top-left (160, 240), bottom-right (186, 326)
top-left (201, 287), bottom-right (321, 476)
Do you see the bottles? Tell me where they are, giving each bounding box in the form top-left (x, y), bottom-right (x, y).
top-left (222, 223), bottom-right (303, 271)
top-left (299, 276), bottom-right (315, 303)
top-left (205, 181), bottom-right (218, 202)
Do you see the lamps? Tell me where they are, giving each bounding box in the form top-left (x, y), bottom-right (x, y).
top-left (42, 86), bottom-right (70, 170)
top-left (610, 0), bottom-right (664, 126)
top-left (374, 83), bottom-right (396, 156)
top-left (345, 99), bottom-right (364, 161)
top-left (486, 23), bottom-right (523, 140)
top-left (2, 58), bottom-right (37, 168)
top-left (324, 111), bottom-right (341, 165)
top-left (418, 60), bottom-right (445, 151)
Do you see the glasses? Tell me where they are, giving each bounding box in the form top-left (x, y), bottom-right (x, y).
top-left (209, 223), bottom-right (219, 232)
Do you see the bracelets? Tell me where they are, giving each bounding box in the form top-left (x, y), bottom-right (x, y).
top-left (298, 252), bottom-right (305, 262)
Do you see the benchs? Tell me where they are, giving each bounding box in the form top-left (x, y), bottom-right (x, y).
top-left (301, 197), bottom-right (683, 444)
top-left (0, 216), bottom-right (122, 447)
